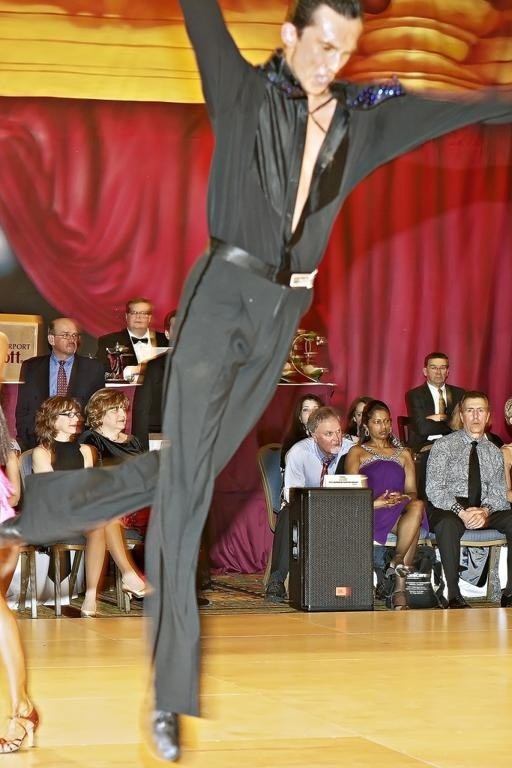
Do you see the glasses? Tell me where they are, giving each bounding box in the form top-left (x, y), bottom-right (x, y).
top-left (59, 411), bottom-right (81, 418)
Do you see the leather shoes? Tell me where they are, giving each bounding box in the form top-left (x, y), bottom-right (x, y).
top-left (0, 516), bottom-right (29, 551)
top-left (499, 593), bottom-right (512, 608)
top-left (153, 710), bottom-right (182, 761)
top-left (448, 595), bottom-right (472, 609)
top-left (81, 600), bottom-right (98, 616)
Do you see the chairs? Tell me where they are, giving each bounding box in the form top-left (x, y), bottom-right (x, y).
top-left (253, 414), bottom-right (512, 606)
top-left (11, 444), bottom-right (147, 616)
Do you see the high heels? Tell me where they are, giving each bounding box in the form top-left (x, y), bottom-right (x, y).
top-left (122, 583), bottom-right (155, 600)
top-left (384, 556), bottom-right (415, 578)
top-left (1, 702), bottom-right (39, 754)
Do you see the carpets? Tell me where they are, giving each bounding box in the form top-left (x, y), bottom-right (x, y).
top-left (17, 569), bottom-right (503, 617)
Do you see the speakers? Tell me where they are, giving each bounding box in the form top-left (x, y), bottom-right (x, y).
top-left (288, 486), bottom-right (375, 612)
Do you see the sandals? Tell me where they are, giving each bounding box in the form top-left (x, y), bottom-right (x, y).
top-left (391, 591), bottom-right (410, 611)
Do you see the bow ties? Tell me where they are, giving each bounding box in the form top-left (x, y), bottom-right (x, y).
top-left (131, 336), bottom-right (149, 344)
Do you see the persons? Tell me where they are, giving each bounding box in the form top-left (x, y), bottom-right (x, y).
top-left (0, 405), bottom-right (39, 755)
top-left (0, 389), bottom-right (155, 618)
top-left (95, 299), bottom-right (177, 449)
top-left (269, 393), bottom-right (512, 608)
top-left (0, 0), bottom-right (512, 764)
top-left (16, 316), bottom-right (106, 452)
top-left (404, 354), bottom-right (464, 501)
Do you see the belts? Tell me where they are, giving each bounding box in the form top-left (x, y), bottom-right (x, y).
top-left (208, 235), bottom-right (320, 293)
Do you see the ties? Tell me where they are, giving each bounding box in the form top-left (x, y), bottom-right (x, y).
top-left (56, 359), bottom-right (69, 397)
top-left (319, 459), bottom-right (332, 488)
top-left (438, 388), bottom-right (447, 415)
top-left (468, 441), bottom-right (482, 507)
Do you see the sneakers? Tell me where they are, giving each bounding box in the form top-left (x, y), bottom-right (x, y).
top-left (267, 581), bottom-right (287, 602)
